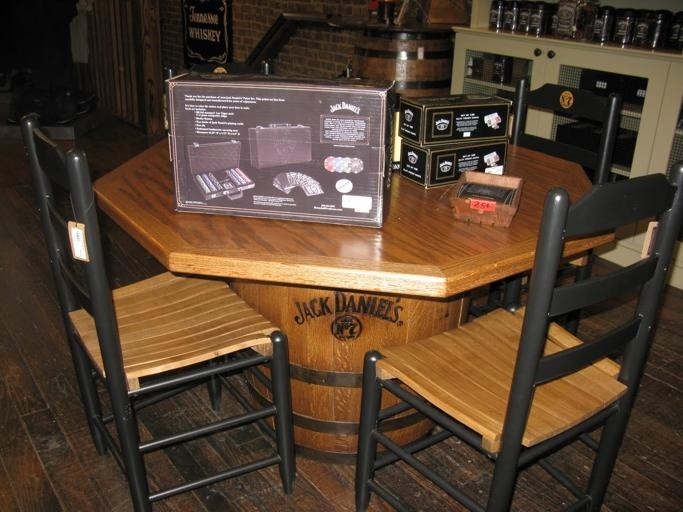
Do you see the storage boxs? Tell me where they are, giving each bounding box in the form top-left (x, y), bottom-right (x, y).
top-left (166, 76), bottom-right (396, 231)
top-left (399, 138), bottom-right (509, 191)
top-left (399, 93), bottom-right (511, 148)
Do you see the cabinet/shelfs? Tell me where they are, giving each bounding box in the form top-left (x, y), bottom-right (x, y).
top-left (446, 24), bottom-right (682, 289)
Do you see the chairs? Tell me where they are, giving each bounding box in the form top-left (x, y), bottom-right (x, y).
top-left (20, 108), bottom-right (298, 510)
top-left (354, 172), bottom-right (682, 512)
top-left (463, 79), bottom-right (625, 332)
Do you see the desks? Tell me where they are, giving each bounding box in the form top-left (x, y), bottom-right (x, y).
top-left (94, 133), bottom-right (614, 468)
